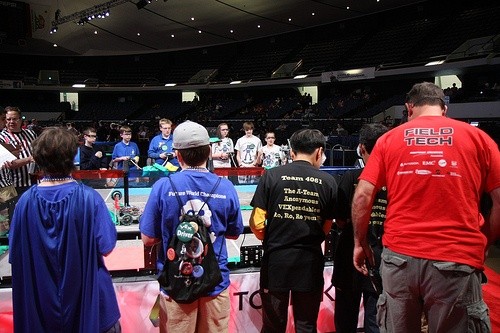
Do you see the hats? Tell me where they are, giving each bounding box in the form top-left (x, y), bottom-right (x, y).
top-left (172, 120), bottom-right (211, 150)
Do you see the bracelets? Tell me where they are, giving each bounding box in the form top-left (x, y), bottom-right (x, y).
top-left (253, 164), bottom-right (256, 166)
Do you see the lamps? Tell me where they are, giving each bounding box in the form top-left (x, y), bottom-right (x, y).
top-left (53, 27), bottom-right (58, 33)
top-left (98, 13), bottom-right (101, 18)
top-left (88, 16), bottom-right (91, 21)
top-left (103, 11), bottom-right (105, 15)
top-left (84, 17), bottom-right (87, 24)
top-left (107, 8), bottom-right (110, 12)
top-left (76, 19), bottom-right (82, 26)
top-left (148, 0), bottom-right (152, 4)
top-left (49, 27), bottom-right (53, 35)
top-left (92, 14), bottom-right (96, 19)
top-left (106, 12), bottom-right (110, 17)
top-left (80, 21), bottom-right (84, 26)
top-left (102, 14), bottom-right (105, 19)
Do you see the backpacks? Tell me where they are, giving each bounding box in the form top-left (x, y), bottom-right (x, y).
top-left (156, 176), bottom-right (224, 305)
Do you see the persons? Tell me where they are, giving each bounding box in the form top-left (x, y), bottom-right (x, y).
top-left (8, 127), bottom-right (122, 333)
top-left (0, 105), bottom-right (44, 200)
top-left (257, 132), bottom-right (287, 177)
top-left (80, 124), bottom-right (107, 189)
top-left (209, 123), bottom-right (234, 179)
top-left (331, 122), bottom-right (389, 333)
top-left (21, 114), bottom-right (160, 142)
top-left (354, 82), bottom-right (500, 333)
top-left (0, 105), bottom-right (35, 246)
top-left (234, 121), bottom-right (263, 184)
top-left (443, 83), bottom-right (462, 98)
top-left (328, 90), bottom-right (379, 113)
top-left (112, 126), bottom-right (139, 188)
top-left (484, 77), bottom-right (496, 89)
top-left (139, 121), bottom-right (244, 333)
top-left (177, 93), bottom-right (320, 139)
top-left (148, 118), bottom-right (182, 188)
top-left (249, 128), bottom-right (338, 333)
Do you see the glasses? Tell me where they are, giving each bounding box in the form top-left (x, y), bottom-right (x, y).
top-left (221, 129), bottom-right (230, 132)
top-left (267, 137), bottom-right (275, 139)
top-left (86, 135), bottom-right (98, 139)
